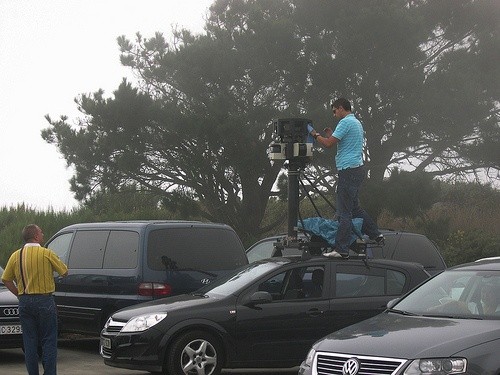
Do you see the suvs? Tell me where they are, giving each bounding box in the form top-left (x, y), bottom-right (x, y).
top-left (245, 227), bottom-right (447, 276)
top-left (297, 257), bottom-right (500, 375)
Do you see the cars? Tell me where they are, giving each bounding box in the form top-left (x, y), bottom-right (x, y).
top-left (100, 255), bottom-right (433, 375)
top-left (0, 266), bottom-right (44, 358)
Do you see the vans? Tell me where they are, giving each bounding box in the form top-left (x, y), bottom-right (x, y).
top-left (44, 219), bottom-right (249, 334)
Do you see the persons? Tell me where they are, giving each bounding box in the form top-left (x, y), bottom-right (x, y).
top-left (1, 224), bottom-right (68, 375)
top-left (307, 97), bottom-right (386, 259)
top-left (439, 280), bottom-right (500, 318)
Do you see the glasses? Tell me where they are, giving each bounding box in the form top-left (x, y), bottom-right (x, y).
top-left (333, 108), bottom-right (338, 114)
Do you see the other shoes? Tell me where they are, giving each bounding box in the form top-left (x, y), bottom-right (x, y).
top-left (368, 234), bottom-right (384, 246)
top-left (322, 248), bottom-right (349, 260)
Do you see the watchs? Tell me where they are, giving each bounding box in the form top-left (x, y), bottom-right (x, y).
top-left (313, 133), bottom-right (321, 140)
